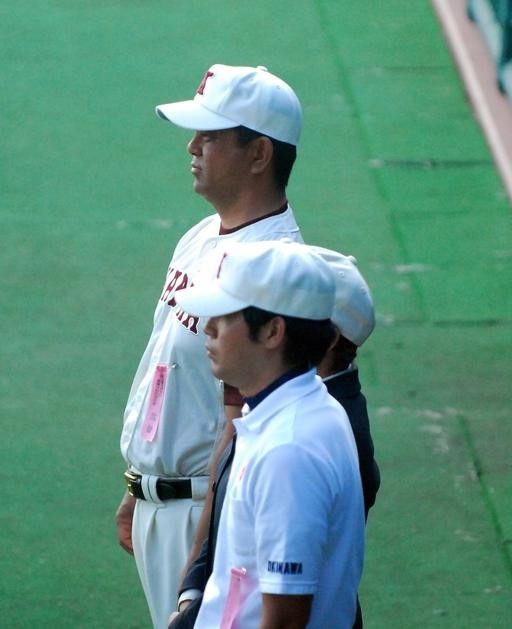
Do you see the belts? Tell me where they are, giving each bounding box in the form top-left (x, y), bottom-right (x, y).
top-left (124, 473), bottom-right (190, 501)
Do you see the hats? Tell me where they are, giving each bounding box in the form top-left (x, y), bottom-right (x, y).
top-left (173, 238), bottom-right (335, 321)
top-left (154, 64), bottom-right (304, 147)
top-left (307, 246), bottom-right (375, 345)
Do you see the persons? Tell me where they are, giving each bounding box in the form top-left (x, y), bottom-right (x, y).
top-left (107, 58), bottom-right (312, 627)
